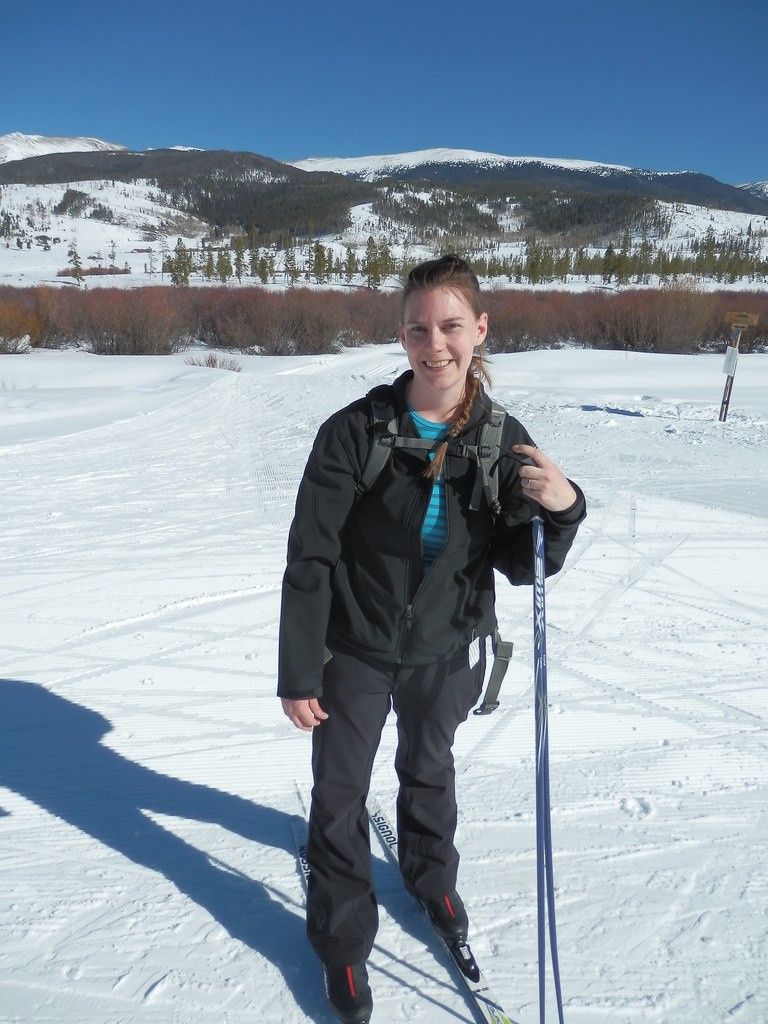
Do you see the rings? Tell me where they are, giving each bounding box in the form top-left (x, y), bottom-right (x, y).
top-left (527, 479), bottom-right (532, 489)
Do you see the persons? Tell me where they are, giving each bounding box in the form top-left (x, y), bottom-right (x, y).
top-left (274, 251), bottom-right (588, 1024)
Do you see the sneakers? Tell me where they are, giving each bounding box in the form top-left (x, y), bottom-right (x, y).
top-left (406, 874), bottom-right (469, 939)
top-left (322, 962), bottom-right (373, 1024)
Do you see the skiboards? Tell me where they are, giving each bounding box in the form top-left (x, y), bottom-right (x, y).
top-left (365, 790), bottom-right (513, 1024)
top-left (291, 812), bottom-right (374, 1024)
